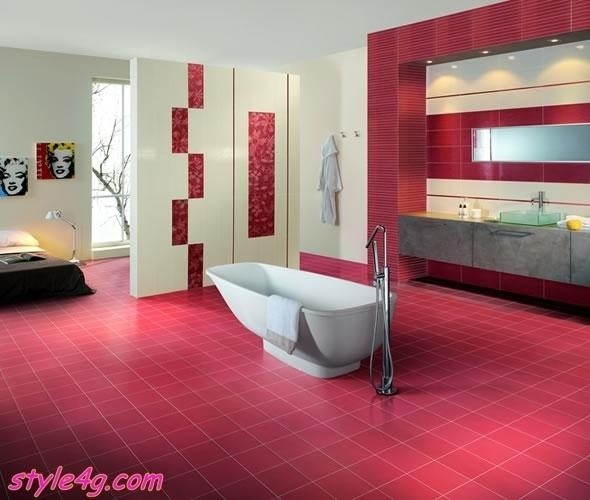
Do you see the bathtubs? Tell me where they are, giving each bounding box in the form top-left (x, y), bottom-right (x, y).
top-left (205, 262), bottom-right (397, 380)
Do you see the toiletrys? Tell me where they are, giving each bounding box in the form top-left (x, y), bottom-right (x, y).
top-left (463, 205), bottom-right (467, 218)
top-left (458, 203), bottom-right (462, 218)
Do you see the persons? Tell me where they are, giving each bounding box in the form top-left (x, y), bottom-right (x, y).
top-left (45, 143), bottom-right (74, 179)
top-left (0, 158), bottom-right (28, 196)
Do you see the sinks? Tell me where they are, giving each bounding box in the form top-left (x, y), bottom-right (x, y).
top-left (499, 209), bottom-right (562, 226)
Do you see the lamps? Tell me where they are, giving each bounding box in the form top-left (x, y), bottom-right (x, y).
top-left (45, 209), bottom-right (79, 264)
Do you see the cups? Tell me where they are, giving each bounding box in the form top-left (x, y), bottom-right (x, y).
top-left (471, 209), bottom-right (481, 218)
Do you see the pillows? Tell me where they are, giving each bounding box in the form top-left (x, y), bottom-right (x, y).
top-left (0, 229), bottom-right (40, 247)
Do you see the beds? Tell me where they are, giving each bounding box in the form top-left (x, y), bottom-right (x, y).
top-left (0, 229), bottom-right (85, 306)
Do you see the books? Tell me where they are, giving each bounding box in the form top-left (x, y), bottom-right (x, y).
top-left (0, 251), bottom-right (48, 265)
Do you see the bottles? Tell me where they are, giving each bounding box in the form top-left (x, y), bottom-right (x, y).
top-left (458, 204), bottom-right (468, 219)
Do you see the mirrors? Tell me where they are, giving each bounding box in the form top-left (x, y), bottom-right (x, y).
top-left (471, 122), bottom-right (590, 164)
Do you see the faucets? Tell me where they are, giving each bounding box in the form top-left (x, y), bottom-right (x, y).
top-left (530, 190), bottom-right (545, 209)
top-left (365, 224), bottom-right (400, 395)
top-left (537, 210), bottom-right (541, 224)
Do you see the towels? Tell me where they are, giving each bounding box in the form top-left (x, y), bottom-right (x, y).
top-left (557, 215), bottom-right (590, 231)
top-left (264, 294), bottom-right (305, 355)
